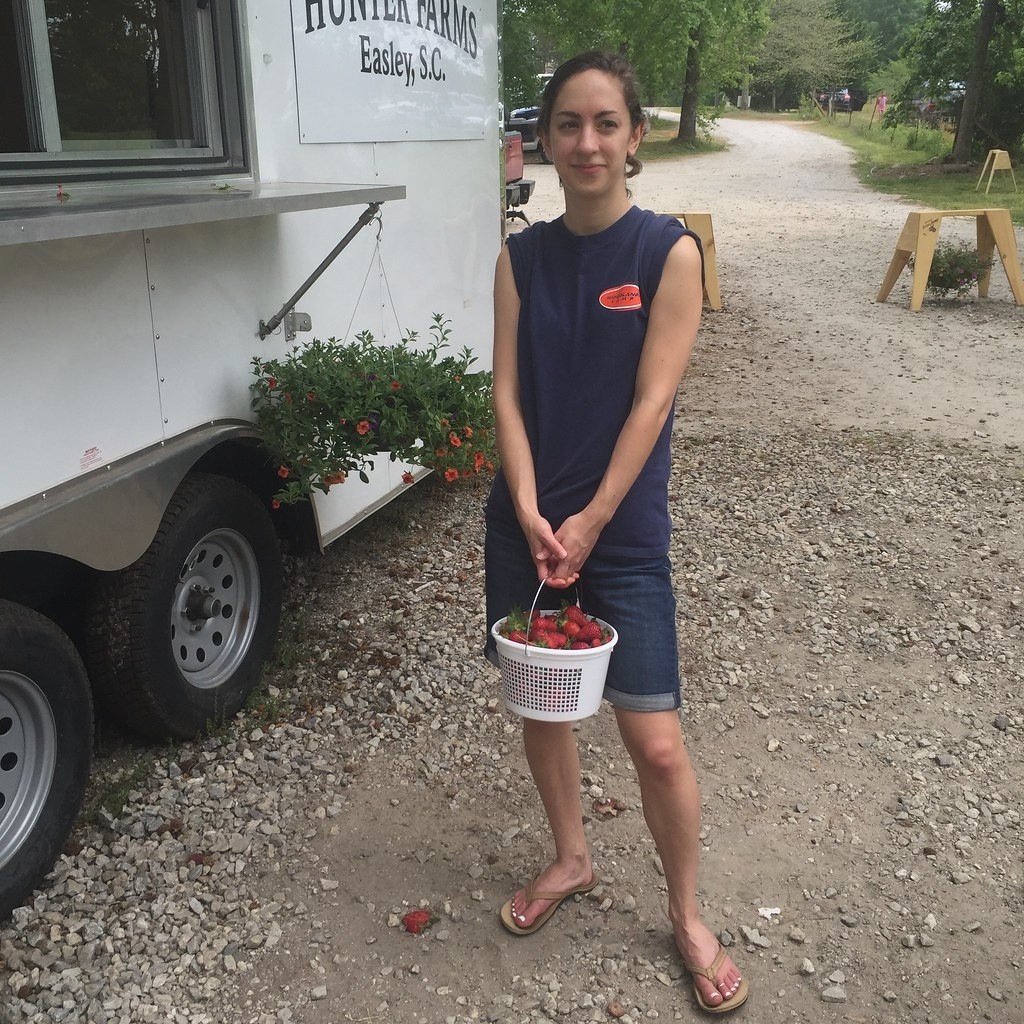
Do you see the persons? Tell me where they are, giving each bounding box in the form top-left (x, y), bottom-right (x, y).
top-left (484, 49), bottom-right (749, 1014)
top-left (877, 92), bottom-right (888, 115)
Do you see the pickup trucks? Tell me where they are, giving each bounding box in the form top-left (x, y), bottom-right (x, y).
top-left (506, 129), bottom-right (536, 227)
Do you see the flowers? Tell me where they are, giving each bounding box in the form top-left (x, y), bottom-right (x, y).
top-left (907, 234), bottom-right (998, 299)
top-left (249, 312), bottom-right (496, 507)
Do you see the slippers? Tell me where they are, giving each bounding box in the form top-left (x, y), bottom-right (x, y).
top-left (683, 940), bottom-right (748, 1014)
top-left (500, 870), bottom-right (599, 934)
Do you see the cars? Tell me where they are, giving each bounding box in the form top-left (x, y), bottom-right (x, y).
top-left (913, 76), bottom-right (967, 132)
top-left (505, 73), bottom-right (554, 166)
top-left (819, 87), bottom-right (851, 112)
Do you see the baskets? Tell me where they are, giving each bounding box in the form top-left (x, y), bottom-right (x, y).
top-left (491, 576), bottom-right (619, 723)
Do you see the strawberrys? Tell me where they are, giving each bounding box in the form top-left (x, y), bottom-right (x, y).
top-left (496, 598), bottom-right (612, 650)
top-left (401, 910), bottom-right (440, 935)
